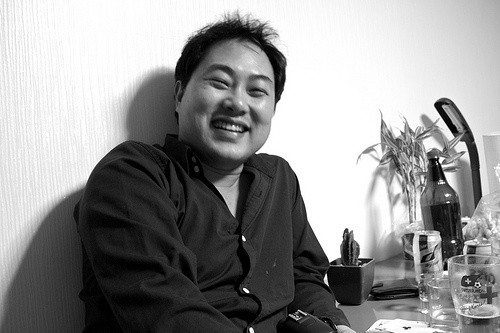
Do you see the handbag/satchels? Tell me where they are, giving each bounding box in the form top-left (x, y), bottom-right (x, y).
top-left (276, 309), bottom-right (338, 333)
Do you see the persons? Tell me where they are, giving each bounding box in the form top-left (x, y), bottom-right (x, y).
top-left (73, 16), bottom-right (358, 333)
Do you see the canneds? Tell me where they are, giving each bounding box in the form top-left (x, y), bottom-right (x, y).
top-left (413, 230), bottom-right (443, 283)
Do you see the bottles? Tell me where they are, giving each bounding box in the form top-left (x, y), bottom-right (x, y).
top-left (420, 151), bottom-right (464, 271)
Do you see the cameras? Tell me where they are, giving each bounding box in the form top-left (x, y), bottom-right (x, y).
top-left (277, 309), bottom-right (337, 333)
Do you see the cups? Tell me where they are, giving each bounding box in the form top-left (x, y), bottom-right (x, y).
top-left (418, 273), bottom-right (443, 303)
top-left (447, 254), bottom-right (500, 333)
top-left (427, 278), bottom-right (459, 327)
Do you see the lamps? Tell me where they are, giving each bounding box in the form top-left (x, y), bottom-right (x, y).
top-left (434, 98), bottom-right (482, 208)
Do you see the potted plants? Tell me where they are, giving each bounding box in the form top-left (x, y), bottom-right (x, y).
top-left (326, 228), bottom-right (375, 305)
top-left (355, 109), bottom-right (466, 266)
